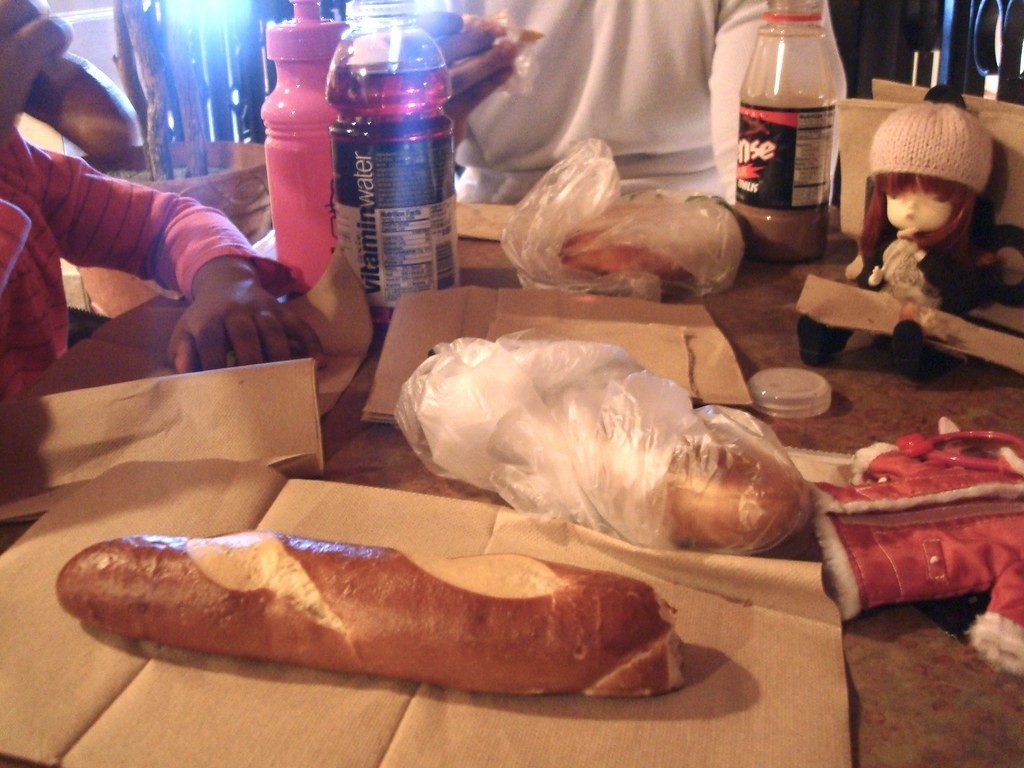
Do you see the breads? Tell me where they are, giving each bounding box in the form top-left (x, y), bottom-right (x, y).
top-left (658, 436), bottom-right (810, 556)
top-left (559, 197), bottom-right (718, 294)
top-left (57, 530), bottom-right (683, 696)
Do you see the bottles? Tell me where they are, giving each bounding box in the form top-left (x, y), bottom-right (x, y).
top-left (252, 0), bottom-right (347, 292)
top-left (322, 0), bottom-right (451, 343)
top-left (732, 0), bottom-right (836, 262)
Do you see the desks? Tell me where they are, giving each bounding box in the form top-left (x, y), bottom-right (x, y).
top-left (0, 199), bottom-right (1024, 768)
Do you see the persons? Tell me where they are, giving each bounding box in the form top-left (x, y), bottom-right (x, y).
top-left (421, 0), bottom-right (849, 210)
top-left (794, 81), bottom-right (1004, 379)
top-left (0, 0), bottom-right (329, 397)
